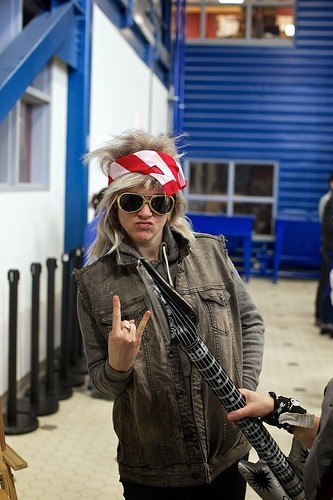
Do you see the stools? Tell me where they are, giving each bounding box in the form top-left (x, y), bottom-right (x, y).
top-left (251, 234), bottom-right (275, 278)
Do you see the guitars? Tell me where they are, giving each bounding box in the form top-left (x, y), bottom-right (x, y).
top-left (136, 258), bottom-right (310, 500)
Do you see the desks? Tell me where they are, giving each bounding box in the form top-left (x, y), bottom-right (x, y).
top-left (275, 219), bottom-right (322, 283)
top-left (185, 212), bottom-right (254, 282)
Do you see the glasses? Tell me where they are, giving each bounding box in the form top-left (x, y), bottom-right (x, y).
top-left (117, 193), bottom-right (175, 216)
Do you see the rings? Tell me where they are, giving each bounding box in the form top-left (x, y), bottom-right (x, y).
top-left (121, 324), bottom-right (131, 333)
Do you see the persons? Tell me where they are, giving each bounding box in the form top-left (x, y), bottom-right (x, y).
top-left (226, 378), bottom-right (333, 500)
top-left (75, 130), bottom-right (265, 500)
top-left (314, 172), bottom-right (333, 335)
